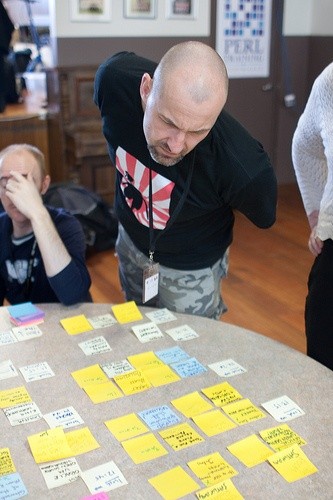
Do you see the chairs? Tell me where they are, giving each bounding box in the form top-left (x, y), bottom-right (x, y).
top-left (60, 67), bottom-right (118, 207)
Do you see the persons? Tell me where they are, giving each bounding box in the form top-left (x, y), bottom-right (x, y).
top-left (0, 143), bottom-right (93, 306)
top-left (292, 61), bottom-right (333, 372)
top-left (92, 40), bottom-right (278, 319)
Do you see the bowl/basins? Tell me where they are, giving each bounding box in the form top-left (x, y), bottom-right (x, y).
top-left (23, 73), bottom-right (47, 101)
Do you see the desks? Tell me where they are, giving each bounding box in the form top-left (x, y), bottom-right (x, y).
top-left (0, 99), bottom-right (52, 175)
top-left (0, 301), bottom-right (333, 499)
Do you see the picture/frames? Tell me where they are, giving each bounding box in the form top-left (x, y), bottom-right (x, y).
top-left (70, 0), bottom-right (112, 22)
top-left (166, 0), bottom-right (196, 19)
top-left (123, 0), bottom-right (159, 19)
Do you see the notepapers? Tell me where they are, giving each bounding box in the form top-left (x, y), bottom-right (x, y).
top-left (8, 301), bottom-right (45, 328)
